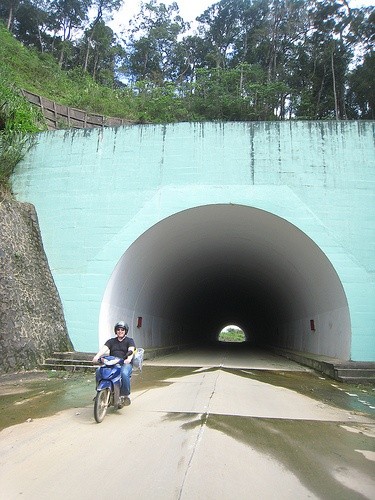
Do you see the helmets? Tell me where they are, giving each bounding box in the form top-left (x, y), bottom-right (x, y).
top-left (114, 321), bottom-right (128, 335)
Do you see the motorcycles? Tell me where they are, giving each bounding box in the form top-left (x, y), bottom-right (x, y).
top-left (91, 355), bottom-right (131, 421)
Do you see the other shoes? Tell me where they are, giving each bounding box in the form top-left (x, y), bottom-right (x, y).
top-left (124, 397), bottom-right (131, 406)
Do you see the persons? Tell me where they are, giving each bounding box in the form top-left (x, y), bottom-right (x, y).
top-left (92, 321), bottom-right (136, 407)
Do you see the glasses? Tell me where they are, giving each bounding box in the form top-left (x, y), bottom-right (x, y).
top-left (117, 328), bottom-right (124, 331)
